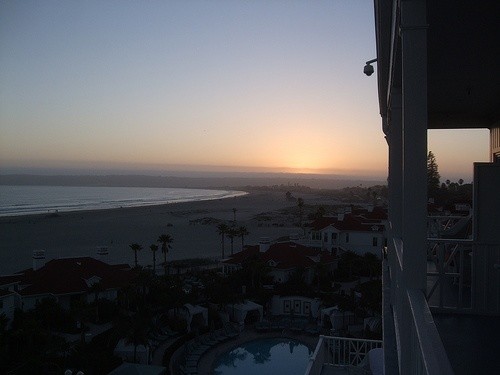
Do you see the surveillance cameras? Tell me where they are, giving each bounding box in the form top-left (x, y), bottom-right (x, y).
top-left (364, 65), bottom-right (374, 75)
